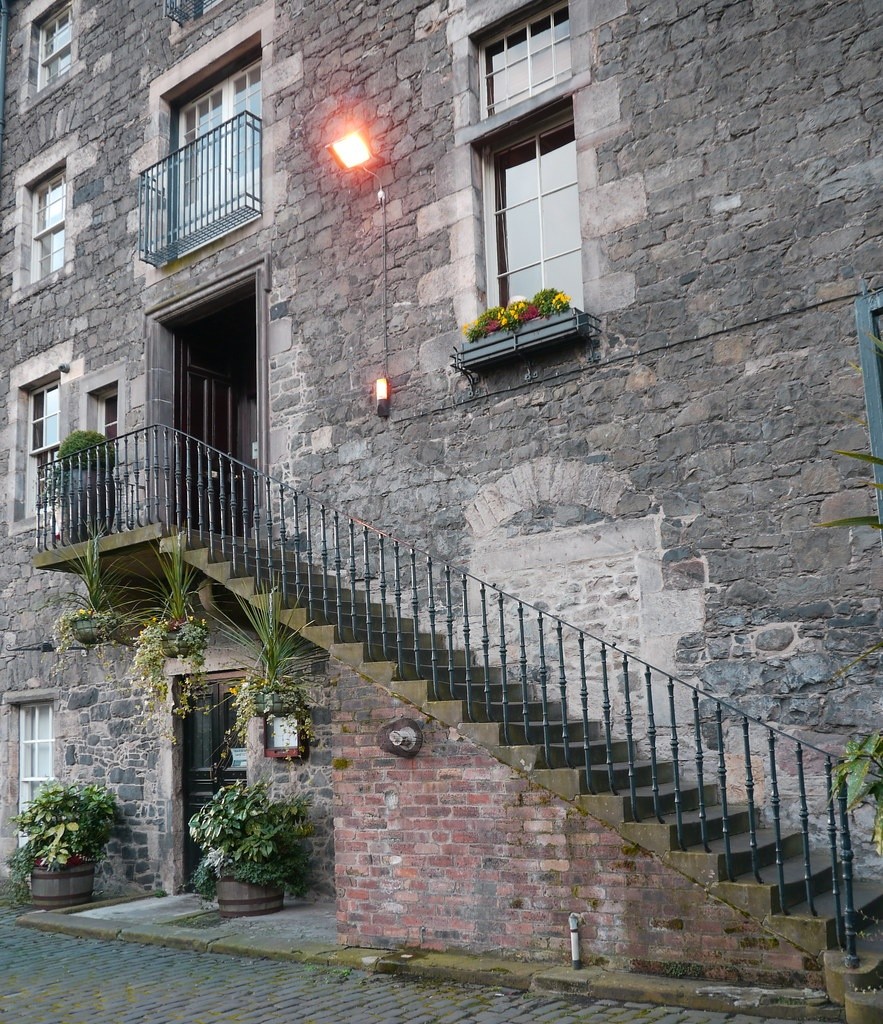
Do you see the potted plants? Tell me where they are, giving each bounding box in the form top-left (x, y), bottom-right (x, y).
top-left (43, 430), bottom-right (115, 543)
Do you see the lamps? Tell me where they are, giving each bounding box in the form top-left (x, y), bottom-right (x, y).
top-left (376, 379), bottom-right (390, 418)
top-left (323, 128), bottom-right (385, 172)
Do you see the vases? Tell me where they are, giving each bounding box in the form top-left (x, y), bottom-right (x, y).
top-left (160, 629), bottom-right (195, 658)
top-left (216, 877), bottom-right (284, 918)
top-left (32, 864), bottom-right (94, 911)
top-left (462, 307), bottom-right (590, 373)
top-left (71, 616), bottom-right (117, 643)
top-left (252, 691), bottom-right (299, 718)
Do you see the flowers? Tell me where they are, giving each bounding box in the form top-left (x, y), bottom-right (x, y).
top-left (463, 287), bottom-right (573, 343)
top-left (46, 517), bottom-right (331, 770)
top-left (188, 779), bottom-right (315, 909)
top-left (3, 778), bottom-right (127, 905)
